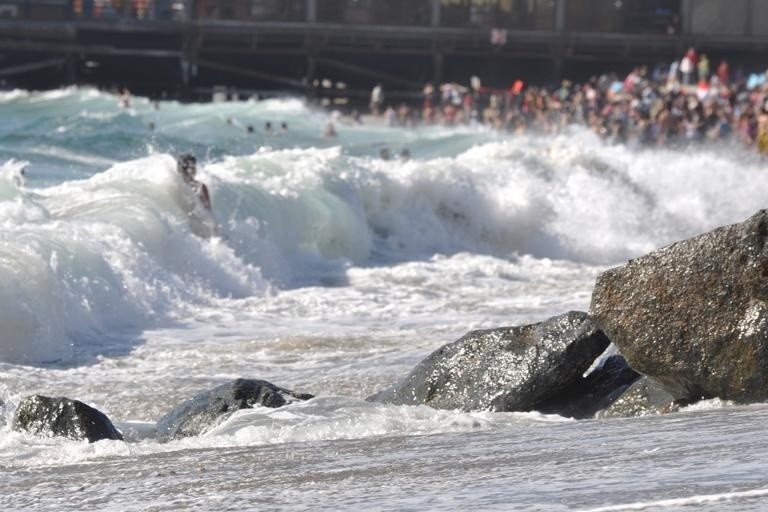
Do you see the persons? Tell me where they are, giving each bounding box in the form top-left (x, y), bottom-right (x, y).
top-left (120, 85), bottom-right (338, 138)
top-left (365, 47), bottom-right (768, 163)
top-left (178, 152), bottom-right (208, 206)
